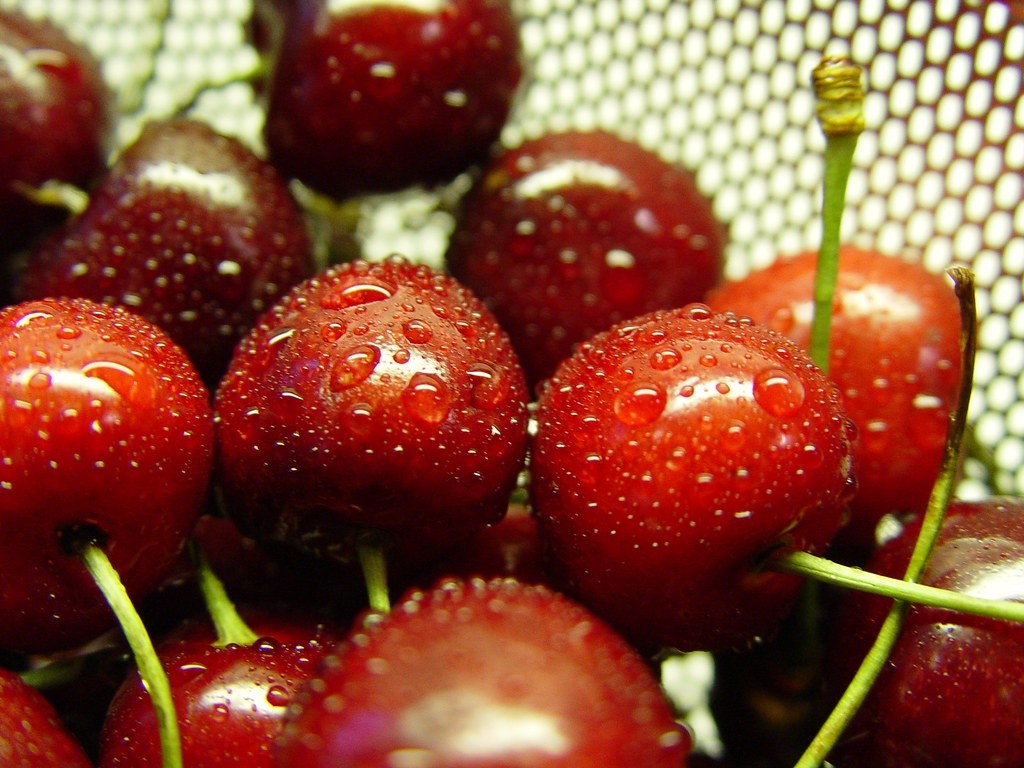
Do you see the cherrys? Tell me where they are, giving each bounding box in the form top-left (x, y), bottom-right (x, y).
top-left (1, 0), bottom-right (1024, 768)
